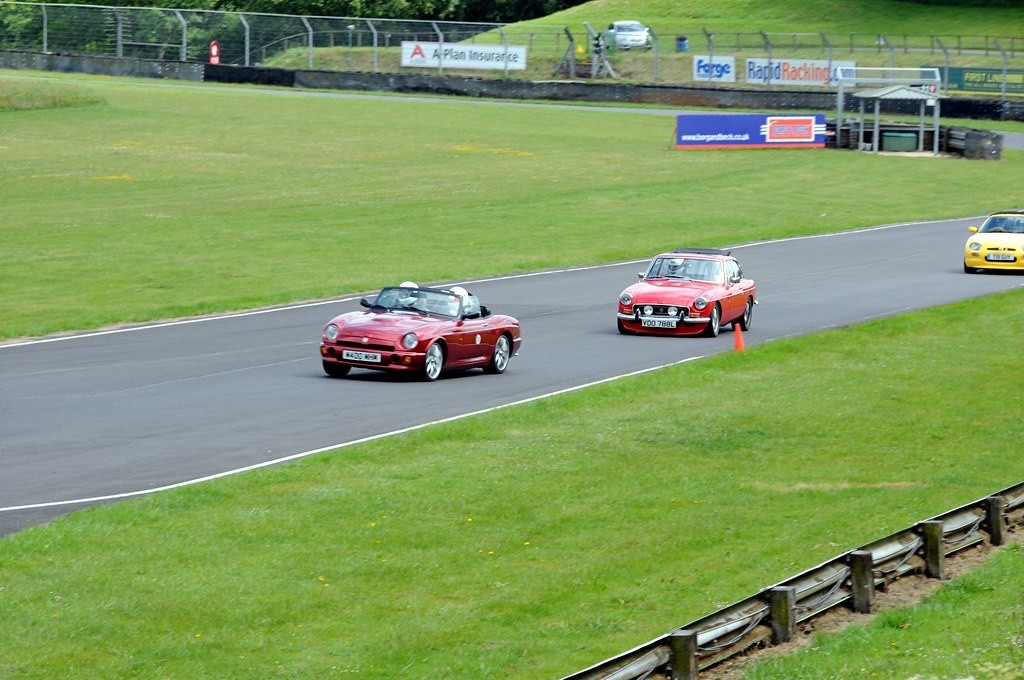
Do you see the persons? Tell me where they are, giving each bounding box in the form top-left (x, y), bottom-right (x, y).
top-left (391, 281), bottom-right (474, 316)
top-left (663, 258), bottom-right (691, 280)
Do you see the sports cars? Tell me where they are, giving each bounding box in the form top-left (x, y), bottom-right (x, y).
top-left (615, 248), bottom-right (760, 338)
top-left (320, 284), bottom-right (523, 382)
top-left (963, 209), bottom-right (1024, 274)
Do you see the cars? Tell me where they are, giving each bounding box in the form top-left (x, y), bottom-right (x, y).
top-left (605, 20), bottom-right (653, 50)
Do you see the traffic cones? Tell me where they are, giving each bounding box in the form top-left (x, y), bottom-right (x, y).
top-left (734, 323), bottom-right (746, 353)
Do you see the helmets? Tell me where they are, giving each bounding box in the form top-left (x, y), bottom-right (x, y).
top-left (667, 259), bottom-right (686, 276)
top-left (398, 281), bottom-right (419, 305)
top-left (448, 287), bottom-right (468, 310)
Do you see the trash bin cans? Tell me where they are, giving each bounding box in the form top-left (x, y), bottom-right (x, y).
top-left (676, 36), bottom-right (688, 52)
